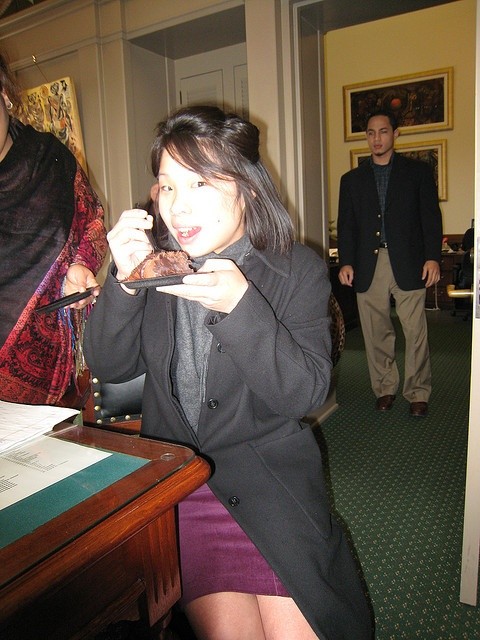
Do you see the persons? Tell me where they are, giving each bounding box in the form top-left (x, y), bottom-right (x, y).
top-left (2, 49), bottom-right (106, 410)
top-left (82, 106), bottom-right (378, 639)
top-left (337, 110), bottom-right (442, 415)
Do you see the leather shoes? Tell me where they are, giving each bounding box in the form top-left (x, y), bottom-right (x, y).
top-left (410, 401), bottom-right (428, 418)
top-left (377, 395), bottom-right (396, 411)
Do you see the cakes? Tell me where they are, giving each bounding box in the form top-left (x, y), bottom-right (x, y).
top-left (122, 248), bottom-right (194, 282)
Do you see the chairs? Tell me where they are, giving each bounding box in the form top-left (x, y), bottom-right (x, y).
top-left (90, 370), bottom-right (145, 435)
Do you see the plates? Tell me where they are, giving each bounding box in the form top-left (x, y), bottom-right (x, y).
top-left (34, 286), bottom-right (99, 314)
top-left (114, 273), bottom-right (214, 289)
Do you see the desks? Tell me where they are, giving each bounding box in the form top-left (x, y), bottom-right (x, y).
top-left (0, 424), bottom-right (211, 640)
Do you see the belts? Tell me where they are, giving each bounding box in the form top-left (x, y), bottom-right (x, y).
top-left (380, 243), bottom-right (388, 248)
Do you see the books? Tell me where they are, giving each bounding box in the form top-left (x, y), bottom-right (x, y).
top-left (0, 398), bottom-right (151, 552)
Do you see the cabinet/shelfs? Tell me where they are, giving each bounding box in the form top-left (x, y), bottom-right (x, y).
top-left (424, 255), bottom-right (456, 310)
top-left (329, 264), bottom-right (358, 332)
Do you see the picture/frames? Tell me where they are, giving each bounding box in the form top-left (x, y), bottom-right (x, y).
top-left (348, 138), bottom-right (449, 203)
top-left (341, 66), bottom-right (455, 143)
